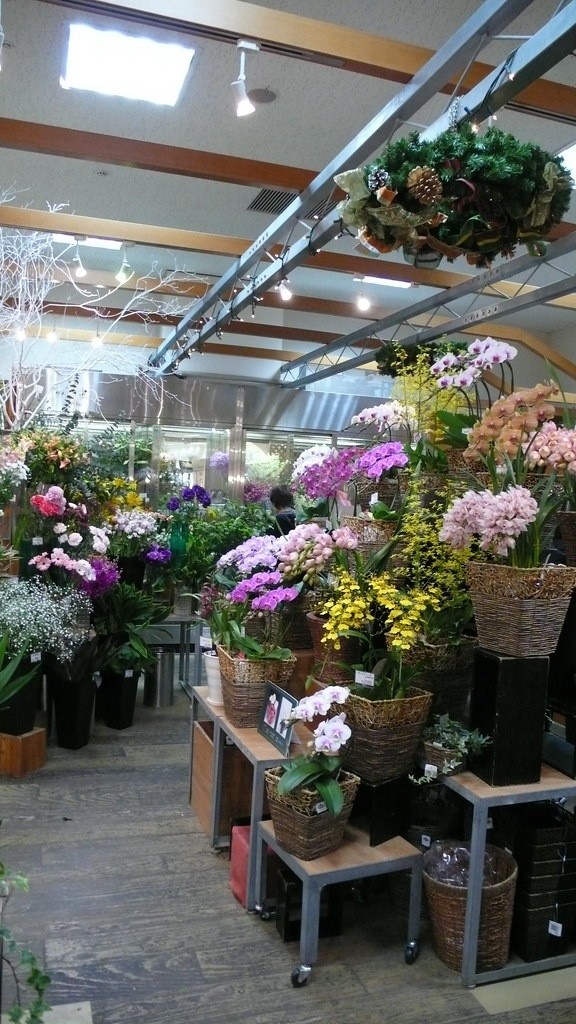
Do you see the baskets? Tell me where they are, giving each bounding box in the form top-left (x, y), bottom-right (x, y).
top-left (264, 766), bottom-right (361, 860)
top-left (516, 473), bottom-right (568, 563)
top-left (216, 643), bottom-right (297, 729)
top-left (417, 740), bottom-right (467, 777)
top-left (219, 449), bottom-right (512, 650)
top-left (305, 611), bottom-right (361, 684)
top-left (422, 841), bottom-right (519, 974)
top-left (384, 633), bottom-right (480, 725)
top-left (556, 511), bottom-right (576, 567)
top-left (327, 678), bottom-right (434, 788)
top-left (387, 801), bottom-right (457, 926)
top-left (467, 561), bottom-right (576, 657)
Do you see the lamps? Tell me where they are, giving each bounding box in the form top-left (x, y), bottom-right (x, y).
top-left (116, 242), bottom-right (136, 282)
top-left (278, 281), bottom-right (292, 301)
top-left (230, 38), bottom-right (261, 117)
top-left (72, 234), bottom-right (87, 278)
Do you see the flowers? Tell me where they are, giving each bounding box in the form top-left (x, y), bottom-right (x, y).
top-left (0, 336), bottom-right (576, 818)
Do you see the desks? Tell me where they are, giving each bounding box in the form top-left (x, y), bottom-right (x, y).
top-left (187, 685), bottom-right (340, 915)
top-left (416, 754), bottom-right (576, 988)
top-left (256, 820), bottom-right (425, 987)
top-left (134, 614), bottom-right (203, 702)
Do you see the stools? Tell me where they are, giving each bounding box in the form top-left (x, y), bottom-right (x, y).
top-left (229, 824), bottom-right (277, 907)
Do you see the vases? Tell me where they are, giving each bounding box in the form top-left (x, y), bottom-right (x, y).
top-left (0, 448), bottom-right (576, 974)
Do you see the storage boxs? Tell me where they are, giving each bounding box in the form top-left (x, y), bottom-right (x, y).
top-left (277, 871), bottom-right (342, 942)
top-left (190, 720), bottom-right (271, 834)
